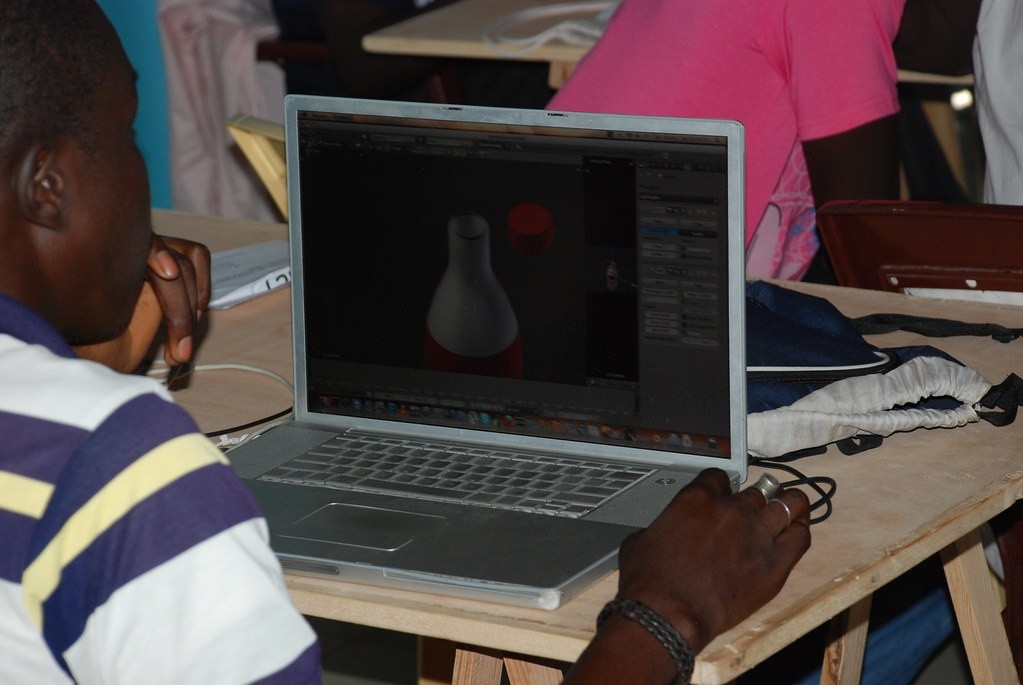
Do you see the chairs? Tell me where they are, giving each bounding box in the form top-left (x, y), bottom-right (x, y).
top-left (815, 198), bottom-right (1023, 303)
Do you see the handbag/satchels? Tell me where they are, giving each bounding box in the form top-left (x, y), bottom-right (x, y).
top-left (743, 279), bottom-right (1023, 462)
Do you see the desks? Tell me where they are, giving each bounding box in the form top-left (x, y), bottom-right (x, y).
top-left (135, 207), bottom-right (1023, 685)
top-left (362, 0), bottom-right (974, 196)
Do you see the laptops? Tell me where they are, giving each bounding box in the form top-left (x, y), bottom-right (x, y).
top-left (221, 95), bottom-right (749, 609)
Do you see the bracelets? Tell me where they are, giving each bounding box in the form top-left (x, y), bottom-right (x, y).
top-left (596, 599), bottom-right (695, 685)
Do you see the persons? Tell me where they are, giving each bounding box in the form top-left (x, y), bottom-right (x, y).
top-left (0, 0), bottom-right (809, 685)
top-left (544, 0), bottom-right (904, 286)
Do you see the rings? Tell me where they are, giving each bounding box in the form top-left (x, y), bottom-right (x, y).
top-left (769, 497), bottom-right (793, 527)
top-left (749, 472), bottom-right (780, 505)
